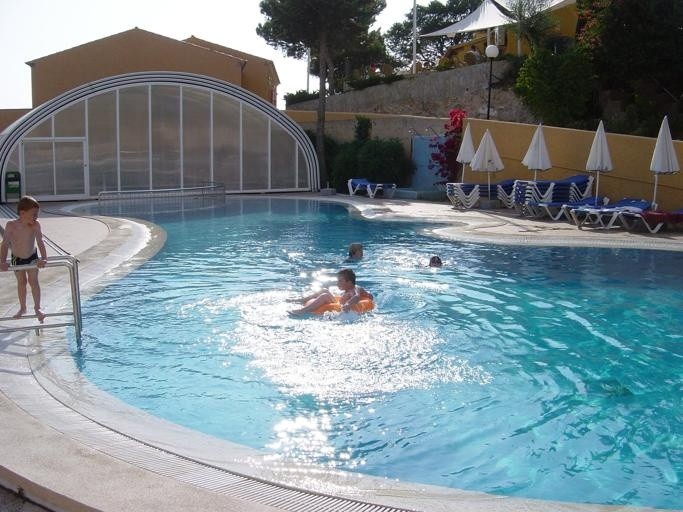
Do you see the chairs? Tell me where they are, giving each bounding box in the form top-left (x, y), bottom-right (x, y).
top-left (447, 172), bottom-right (683, 237)
top-left (348, 178), bottom-right (396, 199)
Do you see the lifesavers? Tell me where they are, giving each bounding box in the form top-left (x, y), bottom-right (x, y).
top-left (304, 295), bottom-right (373, 315)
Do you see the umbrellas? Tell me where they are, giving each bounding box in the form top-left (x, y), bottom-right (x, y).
top-left (519, 122), bottom-right (553, 182)
top-left (453, 122), bottom-right (475, 183)
top-left (469, 128), bottom-right (504, 200)
top-left (583, 118), bottom-right (615, 205)
top-left (648, 115), bottom-right (681, 211)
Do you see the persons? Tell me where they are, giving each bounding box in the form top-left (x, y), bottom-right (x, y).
top-left (283, 268), bottom-right (373, 318)
top-left (0, 193), bottom-right (48, 324)
top-left (332, 243), bottom-right (363, 263)
top-left (417, 254), bottom-right (443, 269)
top-left (467, 45), bottom-right (481, 62)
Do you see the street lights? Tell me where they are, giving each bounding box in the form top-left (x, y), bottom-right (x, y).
top-left (482, 44), bottom-right (499, 119)
